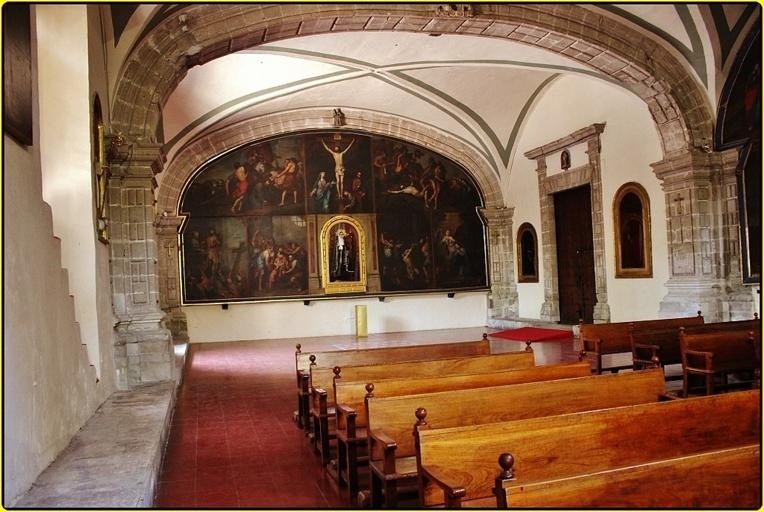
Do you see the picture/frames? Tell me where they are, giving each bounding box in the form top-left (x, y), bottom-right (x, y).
top-left (319, 215), bottom-right (367, 294)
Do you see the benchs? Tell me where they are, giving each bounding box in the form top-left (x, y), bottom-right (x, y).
top-left (627, 312), bottom-right (762, 385)
top-left (364, 356), bottom-right (668, 507)
top-left (332, 350), bottom-right (591, 505)
top-left (489, 442), bottom-right (760, 507)
top-left (576, 313), bottom-right (706, 374)
top-left (412, 389), bottom-right (760, 508)
top-left (677, 326), bottom-right (761, 397)
top-left (309, 338), bottom-right (536, 472)
top-left (291, 332), bottom-right (492, 445)
